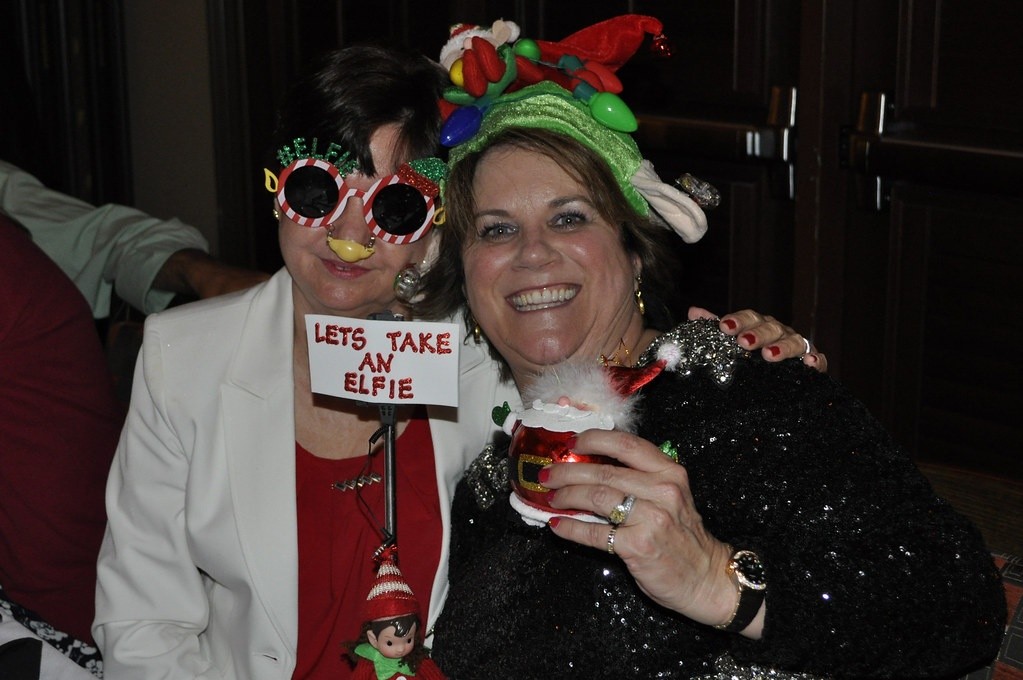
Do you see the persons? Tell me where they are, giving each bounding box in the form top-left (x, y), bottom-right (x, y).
top-left (410, 43), bottom-right (1003, 680)
top-left (90, 37), bottom-right (832, 679)
top-left (353, 541), bottom-right (428, 680)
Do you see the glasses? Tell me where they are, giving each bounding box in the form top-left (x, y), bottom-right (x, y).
top-left (262, 137), bottom-right (448, 244)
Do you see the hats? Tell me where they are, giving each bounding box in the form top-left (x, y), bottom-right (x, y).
top-left (447, 81), bottom-right (721, 247)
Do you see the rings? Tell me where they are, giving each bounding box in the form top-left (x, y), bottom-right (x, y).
top-left (610, 492), bottom-right (637, 526)
top-left (797, 335), bottom-right (812, 364)
top-left (603, 527), bottom-right (619, 555)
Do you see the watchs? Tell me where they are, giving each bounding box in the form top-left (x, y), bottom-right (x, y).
top-left (711, 540), bottom-right (770, 638)
